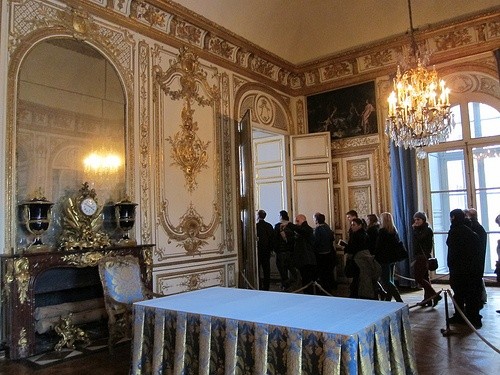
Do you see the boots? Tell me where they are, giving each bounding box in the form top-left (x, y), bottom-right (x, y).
top-left (374, 280), bottom-right (387, 300)
top-left (385, 284), bottom-right (403, 302)
top-left (416, 284), bottom-right (442, 307)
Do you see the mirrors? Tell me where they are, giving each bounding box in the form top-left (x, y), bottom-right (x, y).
top-left (7, 3), bottom-right (134, 250)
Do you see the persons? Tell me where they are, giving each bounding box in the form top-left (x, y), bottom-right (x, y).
top-left (256, 210), bottom-right (274, 291)
top-left (495, 214), bottom-right (500, 313)
top-left (446, 208), bottom-right (487, 328)
top-left (412, 211), bottom-right (442, 307)
top-left (274, 210), bottom-right (403, 302)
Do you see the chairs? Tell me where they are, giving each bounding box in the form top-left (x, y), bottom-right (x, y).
top-left (97, 255), bottom-right (163, 356)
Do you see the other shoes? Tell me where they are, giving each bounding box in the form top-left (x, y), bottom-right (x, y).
top-left (276, 281), bottom-right (291, 292)
top-left (448, 315), bottom-right (482, 327)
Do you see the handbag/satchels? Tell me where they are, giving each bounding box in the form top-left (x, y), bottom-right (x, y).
top-left (343, 259), bottom-right (356, 278)
top-left (395, 240), bottom-right (409, 261)
top-left (427, 258), bottom-right (438, 271)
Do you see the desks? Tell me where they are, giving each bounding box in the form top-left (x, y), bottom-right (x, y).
top-left (130, 285), bottom-right (419, 375)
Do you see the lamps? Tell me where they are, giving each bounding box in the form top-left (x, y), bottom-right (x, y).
top-left (384, 0), bottom-right (456, 159)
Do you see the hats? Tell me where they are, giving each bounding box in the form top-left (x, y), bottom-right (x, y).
top-left (413, 211), bottom-right (426, 221)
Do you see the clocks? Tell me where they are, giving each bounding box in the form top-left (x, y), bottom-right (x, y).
top-left (58, 182), bottom-right (111, 252)
